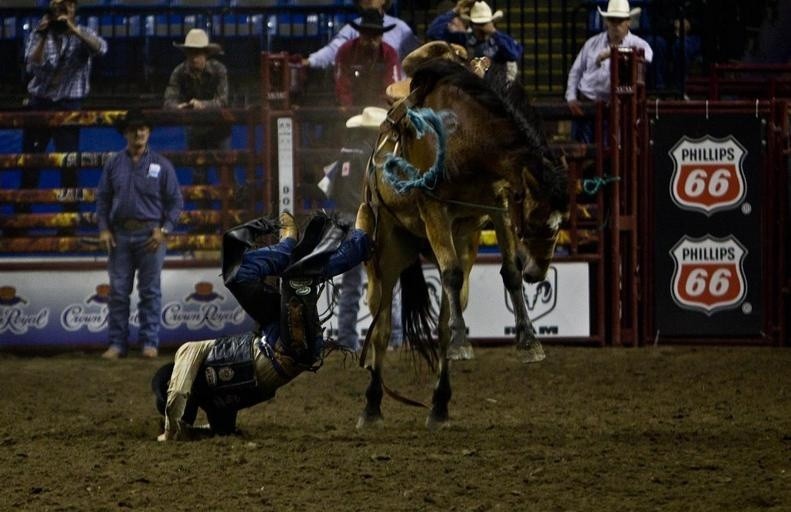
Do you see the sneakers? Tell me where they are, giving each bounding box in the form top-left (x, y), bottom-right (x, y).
top-left (141, 345), bottom-right (158, 358)
top-left (101, 346), bottom-right (122, 360)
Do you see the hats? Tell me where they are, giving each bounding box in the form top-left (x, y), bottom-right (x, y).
top-left (597, 1), bottom-right (642, 18)
top-left (348, 8), bottom-right (396, 32)
top-left (172, 28), bottom-right (222, 58)
top-left (151, 363), bottom-right (198, 426)
top-left (115, 108), bottom-right (152, 133)
top-left (346, 106), bottom-right (389, 131)
top-left (459, 1), bottom-right (504, 24)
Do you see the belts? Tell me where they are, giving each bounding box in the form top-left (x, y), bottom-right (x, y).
top-left (115, 218), bottom-right (153, 231)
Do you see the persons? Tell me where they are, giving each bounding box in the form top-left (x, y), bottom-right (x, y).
top-left (563, 1), bottom-right (656, 204)
top-left (447, 1), bottom-right (521, 92)
top-left (287, 1), bottom-right (424, 83)
top-left (332, 6), bottom-right (403, 110)
top-left (93, 107), bottom-right (185, 360)
top-left (3, 3), bottom-right (110, 237)
top-left (162, 26), bottom-right (251, 236)
top-left (150, 202), bottom-right (378, 444)
top-left (423, 1), bottom-right (475, 44)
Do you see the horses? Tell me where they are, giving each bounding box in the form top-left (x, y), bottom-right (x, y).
top-left (354, 58), bottom-right (578, 435)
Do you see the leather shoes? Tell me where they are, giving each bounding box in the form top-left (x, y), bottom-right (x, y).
top-left (280, 212), bottom-right (299, 241)
top-left (355, 202), bottom-right (376, 259)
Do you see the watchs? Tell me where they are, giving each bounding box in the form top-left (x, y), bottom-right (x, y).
top-left (160, 228), bottom-right (169, 237)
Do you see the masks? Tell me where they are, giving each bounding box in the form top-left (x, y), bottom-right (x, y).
top-left (607, 25), bottom-right (628, 44)
